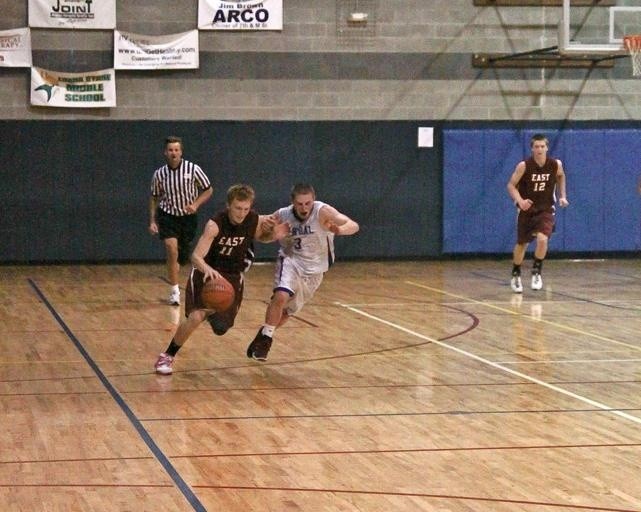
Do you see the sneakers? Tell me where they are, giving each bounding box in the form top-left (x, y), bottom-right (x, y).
top-left (167, 288), bottom-right (181, 305)
top-left (247, 325), bottom-right (272, 361)
top-left (531, 266), bottom-right (543, 289)
top-left (511, 270), bottom-right (523, 293)
top-left (155, 352), bottom-right (175, 375)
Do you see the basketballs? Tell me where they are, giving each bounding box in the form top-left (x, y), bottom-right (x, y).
top-left (201, 278), bottom-right (235, 311)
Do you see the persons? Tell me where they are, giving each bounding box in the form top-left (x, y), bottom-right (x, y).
top-left (506, 133), bottom-right (569, 293)
top-left (247, 182), bottom-right (360, 361)
top-left (147, 135), bottom-right (214, 306)
top-left (153, 182), bottom-right (293, 374)
